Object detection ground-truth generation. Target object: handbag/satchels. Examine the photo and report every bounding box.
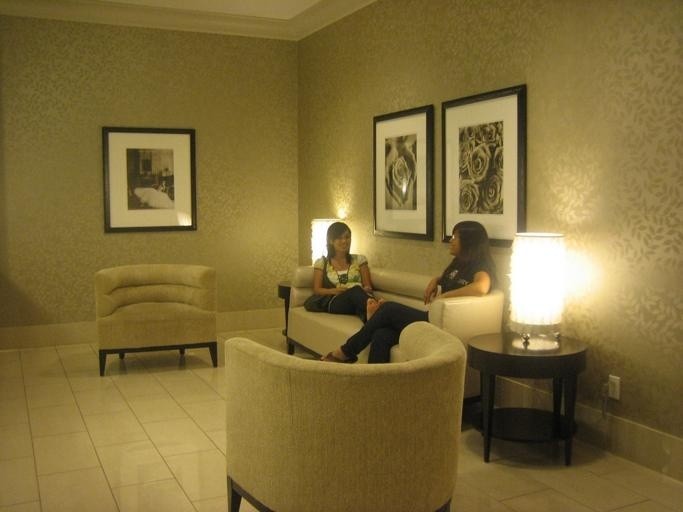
[304,280,336,312]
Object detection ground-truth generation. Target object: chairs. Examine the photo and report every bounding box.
[224,321,466,511]
[93,264,217,377]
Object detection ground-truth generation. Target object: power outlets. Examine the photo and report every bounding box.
[606,375,621,401]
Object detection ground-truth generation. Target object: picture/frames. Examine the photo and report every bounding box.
[372,83,526,249]
[100,125,198,233]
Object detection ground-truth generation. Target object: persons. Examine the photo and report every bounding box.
[318,220,499,368]
[312,221,386,326]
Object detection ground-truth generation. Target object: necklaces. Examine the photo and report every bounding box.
[333,261,349,286]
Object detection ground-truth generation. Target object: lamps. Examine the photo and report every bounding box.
[310,218,339,266]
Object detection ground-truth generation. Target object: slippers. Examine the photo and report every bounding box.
[321,352,357,363]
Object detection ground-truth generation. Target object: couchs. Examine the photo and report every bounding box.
[286,265,505,423]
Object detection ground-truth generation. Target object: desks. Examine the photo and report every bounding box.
[466,333,588,467]
